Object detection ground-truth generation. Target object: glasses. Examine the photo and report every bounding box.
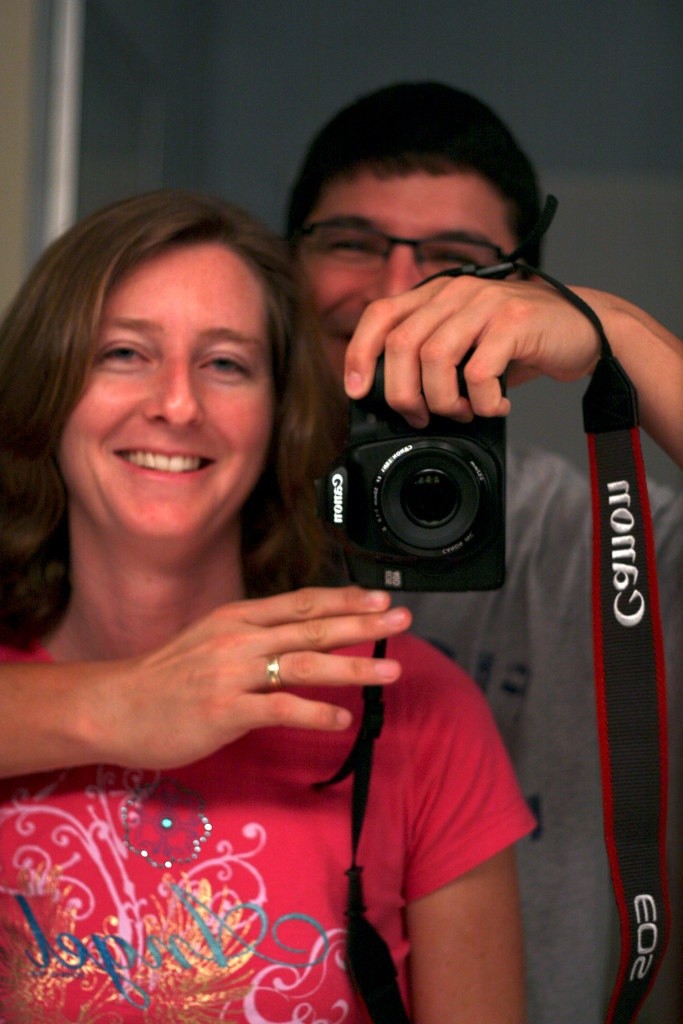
[286,223,515,282]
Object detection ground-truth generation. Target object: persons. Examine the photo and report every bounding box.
[0,80,683,1024]
[0,189,537,1024]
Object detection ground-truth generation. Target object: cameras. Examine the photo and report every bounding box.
[316,354,509,592]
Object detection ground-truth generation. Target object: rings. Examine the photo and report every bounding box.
[262,649,283,695]
